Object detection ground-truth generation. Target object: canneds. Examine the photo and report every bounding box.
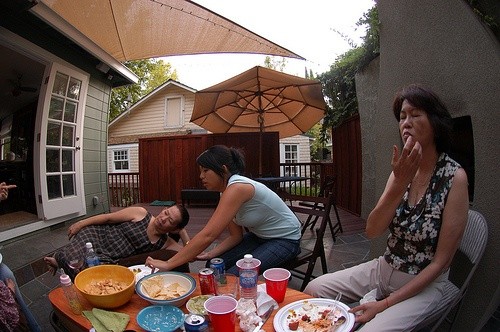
[199,268,217,296]
[210,258,227,287]
[184,314,208,332]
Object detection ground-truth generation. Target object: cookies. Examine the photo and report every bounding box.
[84,279,123,294]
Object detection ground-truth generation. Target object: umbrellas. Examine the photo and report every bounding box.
[191,65,326,178]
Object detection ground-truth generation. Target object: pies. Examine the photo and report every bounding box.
[141,275,188,300]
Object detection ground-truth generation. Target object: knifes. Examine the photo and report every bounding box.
[244,304,274,332]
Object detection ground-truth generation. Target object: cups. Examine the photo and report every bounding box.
[235,258,261,298]
[204,295,238,332]
[263,268,292,303]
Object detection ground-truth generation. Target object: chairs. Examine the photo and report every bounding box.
[301,171,342,234]
[427,210,488,332]
[277,188,334,292]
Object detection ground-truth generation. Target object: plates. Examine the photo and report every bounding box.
[127,263,160,286]
[185,295,215,315]
[272,297,355,332]
[136,305,185,332]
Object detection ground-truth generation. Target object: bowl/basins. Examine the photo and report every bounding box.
[74,265,136,309]
[135,271,197,307]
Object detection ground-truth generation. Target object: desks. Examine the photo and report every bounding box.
[255,176,307,185]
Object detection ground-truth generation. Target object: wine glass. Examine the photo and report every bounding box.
[62,241,84,274]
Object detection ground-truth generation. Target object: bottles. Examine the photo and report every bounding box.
[58,268,82,315]
[238,254,258,303]
[84,242,100,268]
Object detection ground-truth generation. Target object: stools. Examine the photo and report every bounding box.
[117,246,190,272]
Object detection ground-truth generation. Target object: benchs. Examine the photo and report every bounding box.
[179,188,222,207]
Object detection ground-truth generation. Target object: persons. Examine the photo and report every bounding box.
[303,85,470,332]
[0,182,42,332]
[145,145,301,277]
[44,203,190,283]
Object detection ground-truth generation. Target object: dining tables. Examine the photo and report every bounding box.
[48,272,314,332]
